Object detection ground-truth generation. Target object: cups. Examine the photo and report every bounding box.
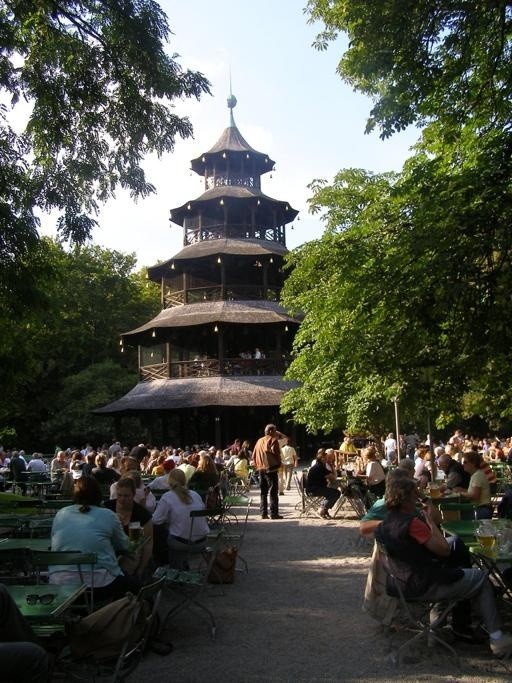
[476,530,495,550]
[128,521,141,543]
[430,485,440,498]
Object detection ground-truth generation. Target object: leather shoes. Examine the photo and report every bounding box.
[271,515,284,520]
[262,514,270,519]
[487,633,512,655]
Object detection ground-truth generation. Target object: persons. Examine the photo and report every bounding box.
[250,424,285,520]
[152,456,166,476]
[52,441,96,490]
[104,479,155,590]
[152,468,211,571]
[207,446,217,462]
[183,446,193,457]
[110,458,157,511]
[1,447,50,498]
[120,443,149,479]
[228,438,241,456]
[392,460,428,502]
[144,449,160,475]
[187,455,220,504]
[454,454,494,520]
[222,448,233,461]
[359,468,477,642]
[144,459,175,491]
[375,478,512,659]
[279,438,297,495]
[225,450,249,493]
[160,443,187,466]
[339,428,512,462]
[49,475,135,604]
[190,445,219,487]
[304,452,342,519]
[325,447,338,477]
[353,444,385,498]
[213,448,225,464]
[237,440,250,465]
[435,454,472,491]
[97,438,129,458]
[92,453,120,499]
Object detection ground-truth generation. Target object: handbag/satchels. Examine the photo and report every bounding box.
[201,532,239,585]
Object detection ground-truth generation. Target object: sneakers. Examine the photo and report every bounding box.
[279,492,285,496]
[320,511,330,518]
[286,485,291,490]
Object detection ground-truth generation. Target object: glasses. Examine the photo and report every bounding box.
[23,592,60,607]
[460,459,473,465]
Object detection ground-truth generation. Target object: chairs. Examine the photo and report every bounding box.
[148,551,216,644]
[172,508,226,596]
[77,572,172,683]
[438,501,476,544]
[231,468,253,496]
[207,499,253,574]
[373,531,465,665]
[290,467,332,519]
